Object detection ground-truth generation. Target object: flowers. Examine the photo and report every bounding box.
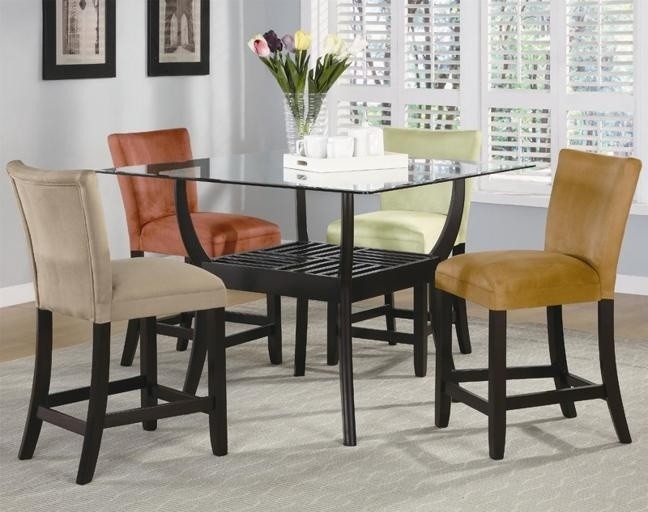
[247,29,368,134]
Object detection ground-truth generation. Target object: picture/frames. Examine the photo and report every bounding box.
[42,0,117,80]
[147,0,211,77]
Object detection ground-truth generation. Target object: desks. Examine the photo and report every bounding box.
[94,153,539,449]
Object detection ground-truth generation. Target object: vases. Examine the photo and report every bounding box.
[283,92,328,152]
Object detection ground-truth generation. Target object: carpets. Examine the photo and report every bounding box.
[0,297,648,512]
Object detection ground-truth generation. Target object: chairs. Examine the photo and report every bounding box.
[433,148,641,462]
[107,127,283,367]
[8,160,228,486]
[325,126,483,376]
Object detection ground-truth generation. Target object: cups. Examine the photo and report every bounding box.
[296,124,384,160]
